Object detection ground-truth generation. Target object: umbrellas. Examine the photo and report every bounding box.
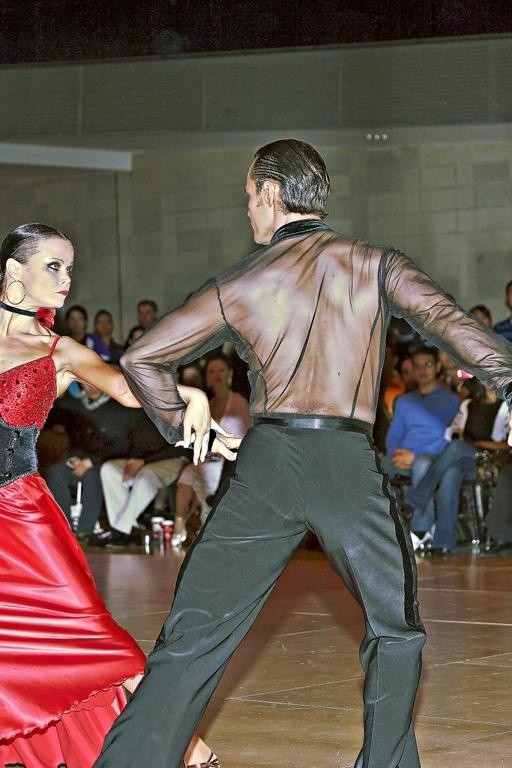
[87,528,189,547]
[398,503,512,558]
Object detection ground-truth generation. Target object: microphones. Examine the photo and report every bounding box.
[385,473,492,556]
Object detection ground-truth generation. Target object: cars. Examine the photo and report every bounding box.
[150,515,174,543]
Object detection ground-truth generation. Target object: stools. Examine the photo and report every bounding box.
[253,417,370,434]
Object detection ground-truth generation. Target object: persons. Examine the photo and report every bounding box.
[97,138,511,768]
[1,222,230,768]
[372,281,510,554]
[37,300,252,546]
